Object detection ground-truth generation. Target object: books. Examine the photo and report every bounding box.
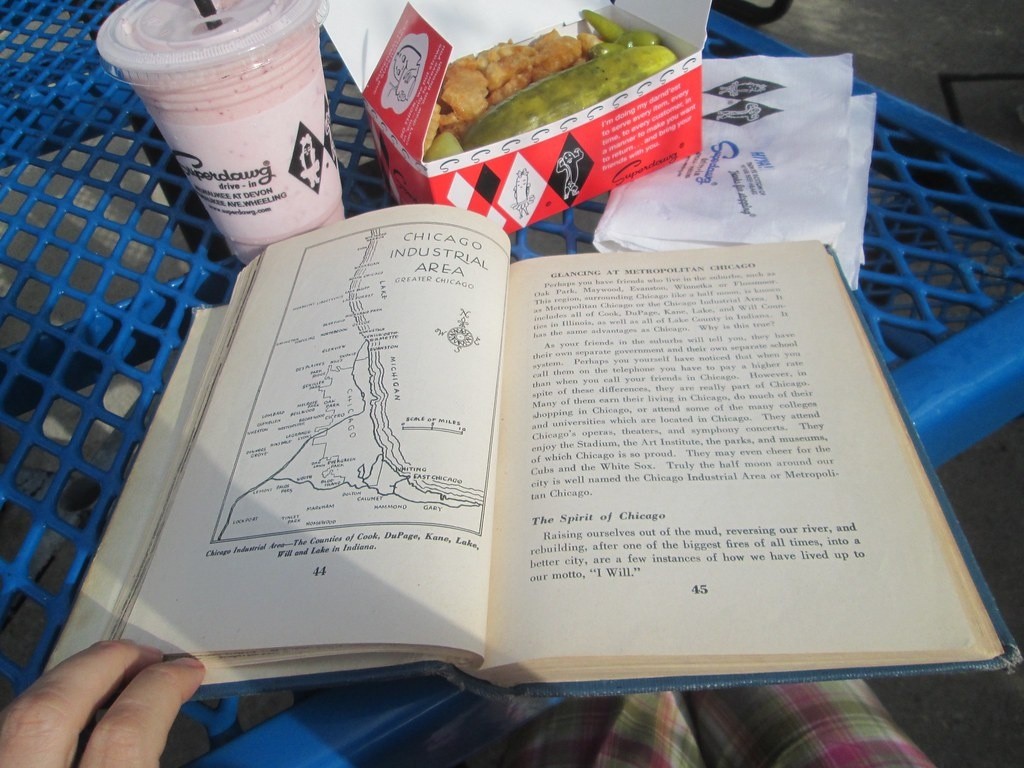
[39,202,1024,704]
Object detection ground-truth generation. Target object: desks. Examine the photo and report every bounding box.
[0,0,1024,768]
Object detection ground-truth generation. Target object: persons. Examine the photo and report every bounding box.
[0,634,941,768]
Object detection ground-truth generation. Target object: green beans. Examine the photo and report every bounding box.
[581,9,660,61]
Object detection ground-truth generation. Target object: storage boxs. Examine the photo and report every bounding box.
[322,0,713,235]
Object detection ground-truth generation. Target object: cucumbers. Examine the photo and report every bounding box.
[458,43,682,153]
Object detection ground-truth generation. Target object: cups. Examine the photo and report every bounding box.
[95,0,346,266]
